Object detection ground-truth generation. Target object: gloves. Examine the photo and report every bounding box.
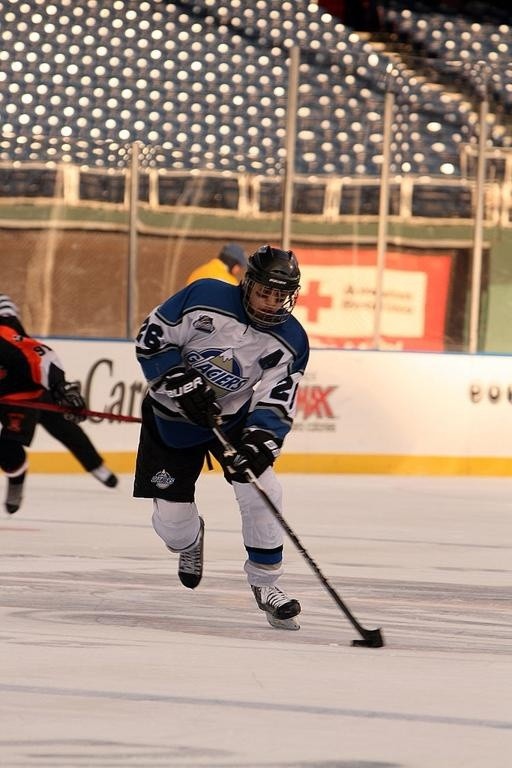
[163,369,227,431]
[221,431,280,484]
[59,383,89,423]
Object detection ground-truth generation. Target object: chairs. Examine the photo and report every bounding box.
[0,1,512,223]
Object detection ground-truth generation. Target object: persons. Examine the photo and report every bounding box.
[132,243,309,619]
[1,292,118,512]
[187,243,248,288]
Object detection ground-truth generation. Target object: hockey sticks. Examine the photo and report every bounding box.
[213,428,390,647]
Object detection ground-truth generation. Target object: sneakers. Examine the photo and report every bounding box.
[90,462,119,489]
[177,517,205,589]
[4,474,26,514]
[251,583,302,619]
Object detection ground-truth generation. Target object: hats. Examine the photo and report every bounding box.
[220,241,247,268]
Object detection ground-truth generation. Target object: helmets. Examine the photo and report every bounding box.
[242,244,302,329]
